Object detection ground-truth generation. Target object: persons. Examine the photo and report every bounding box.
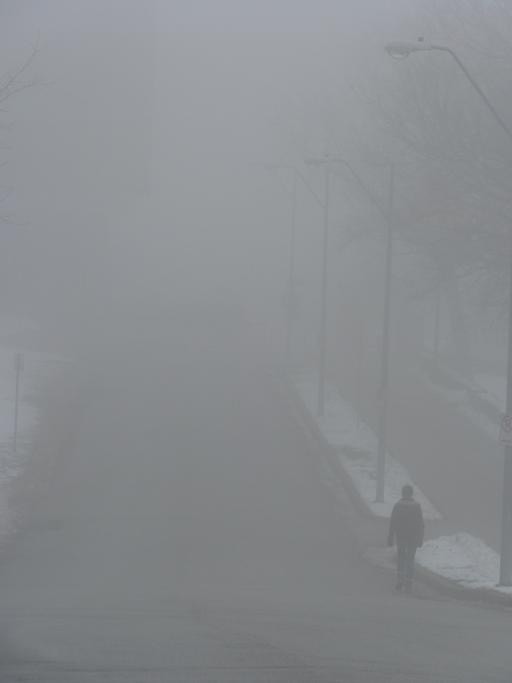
[387,486,425,588]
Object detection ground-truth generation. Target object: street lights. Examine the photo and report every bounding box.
[303,153,396,506]
